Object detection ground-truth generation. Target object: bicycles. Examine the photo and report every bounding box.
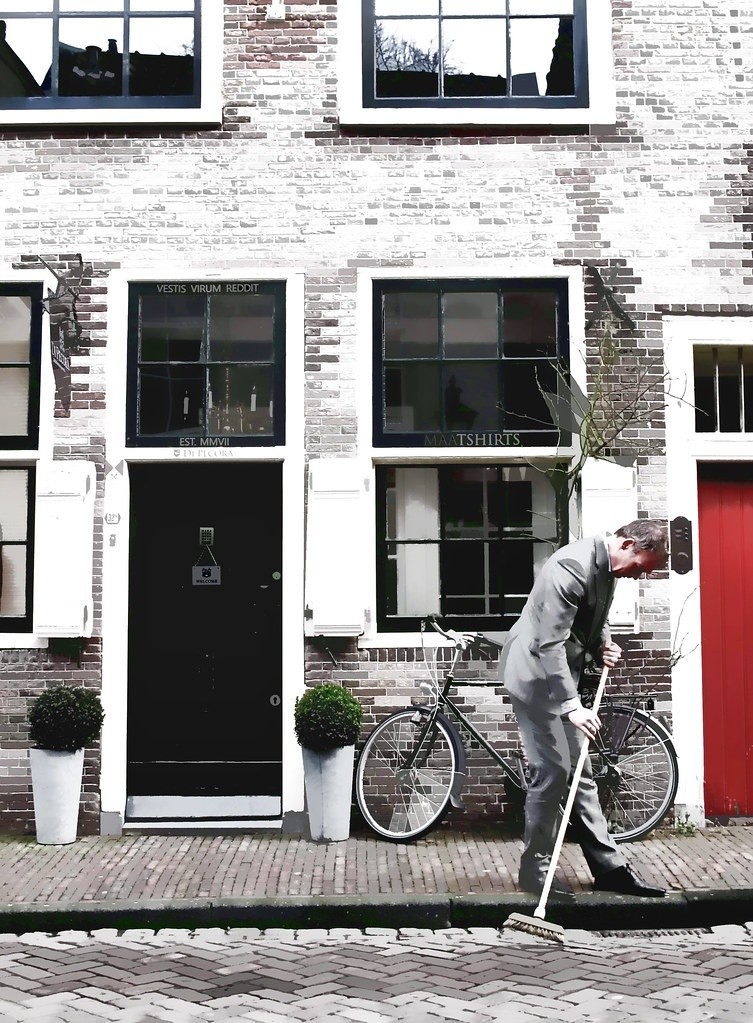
[354,612,681,844]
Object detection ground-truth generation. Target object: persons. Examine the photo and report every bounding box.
[494,517,669,903]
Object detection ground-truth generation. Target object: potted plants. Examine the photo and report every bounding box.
[293,682,364,842]
[26,684,107,844]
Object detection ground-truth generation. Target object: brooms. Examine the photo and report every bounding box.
[501,664,614,947]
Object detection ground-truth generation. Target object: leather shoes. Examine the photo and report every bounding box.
[516,879,578,902]
[593,863,668,897]
